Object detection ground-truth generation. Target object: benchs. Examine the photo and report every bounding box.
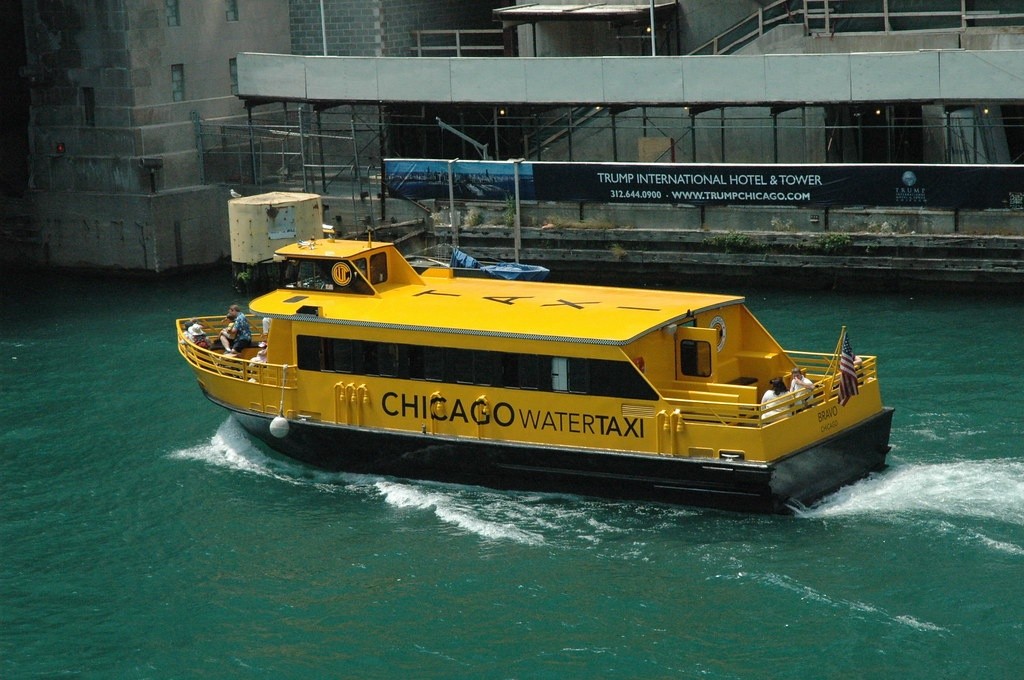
[208,340,267,351]
[724,377,759,386]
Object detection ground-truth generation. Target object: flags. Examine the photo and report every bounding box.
[838,330,859,407]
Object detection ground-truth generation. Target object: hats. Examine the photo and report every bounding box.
[188,323,204,336]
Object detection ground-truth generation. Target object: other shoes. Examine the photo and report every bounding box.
[223,350,238,355]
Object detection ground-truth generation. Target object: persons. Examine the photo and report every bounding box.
[185,318,211,360]
[853,355,863,385]
[239,317,272,382]
[761,378,795,428]
[218,305,252,367]
[217,314,237,341]
[789,368,815,416]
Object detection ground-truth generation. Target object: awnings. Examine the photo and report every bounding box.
[495,3,677,22]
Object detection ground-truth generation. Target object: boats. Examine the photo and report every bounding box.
[174,235,897,515]
[404,241,551,276]
